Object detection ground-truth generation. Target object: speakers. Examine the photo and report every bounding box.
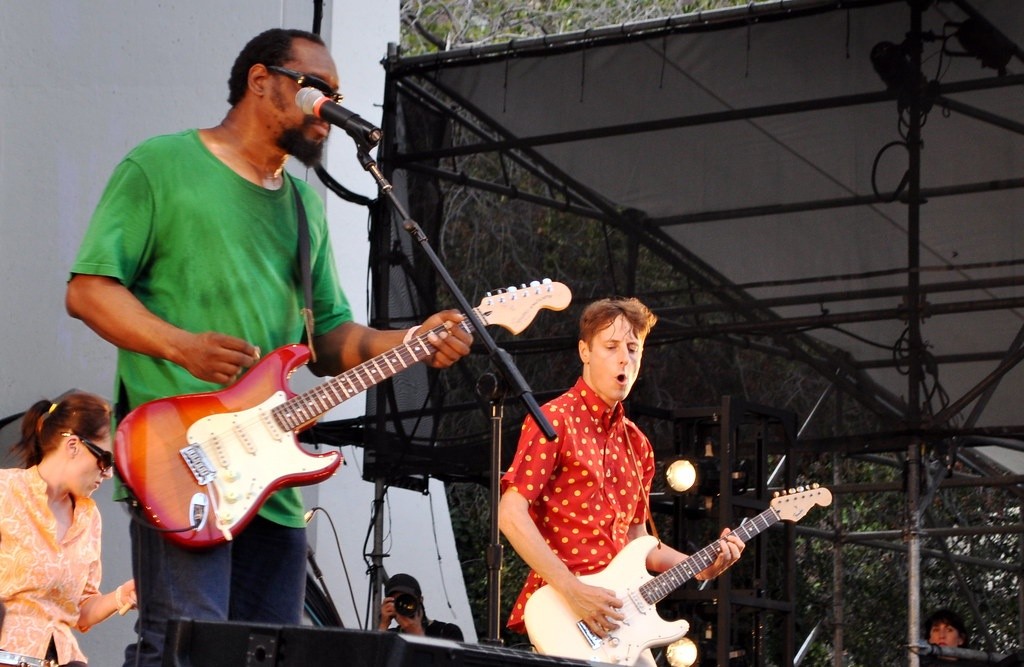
[161,617,626,667]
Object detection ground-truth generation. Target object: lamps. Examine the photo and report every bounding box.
[662,457,718,500]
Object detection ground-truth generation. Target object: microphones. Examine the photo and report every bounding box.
[296,87,381,145]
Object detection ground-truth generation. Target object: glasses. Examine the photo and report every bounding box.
[267,66,343,102]
[60,432,115,472]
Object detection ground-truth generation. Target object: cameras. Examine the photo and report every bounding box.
[393,592,418,617]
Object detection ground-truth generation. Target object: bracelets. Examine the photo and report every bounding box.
[116,585,129,613]
[402,324,421,344]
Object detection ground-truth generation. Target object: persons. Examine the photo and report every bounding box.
[377,573,464,642]
[0,388,112,667]
[63,28,474,667]
[497,295,746,667]
[919,610,969,667]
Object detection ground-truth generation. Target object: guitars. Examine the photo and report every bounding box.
[522,482,833,667]
[112,276,573,550]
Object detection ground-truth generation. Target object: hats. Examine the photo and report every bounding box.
[384,573,421,599]
